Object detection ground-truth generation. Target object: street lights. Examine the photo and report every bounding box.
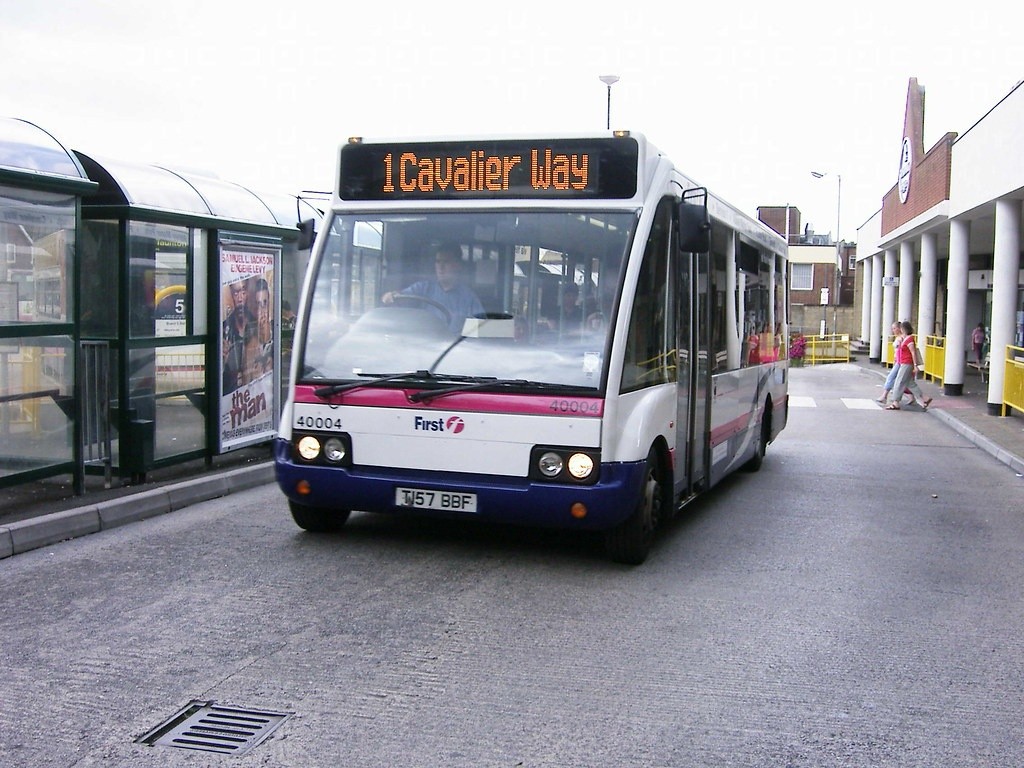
[600,73,620,129]
[811,168,843,359]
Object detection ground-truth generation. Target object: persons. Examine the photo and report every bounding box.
[223,275,275,395]
[383,241,488,339]
[282,300,297,392]
[514,315,528,343]
[745,322,783,367]
[972,323,985,369]
[547,282,605,341]
[876,321,932,410]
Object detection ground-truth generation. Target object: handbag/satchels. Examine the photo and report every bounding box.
[915,348,924,365]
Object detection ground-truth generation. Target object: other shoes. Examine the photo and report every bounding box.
[876,397,887,404]
[906,398,916,405]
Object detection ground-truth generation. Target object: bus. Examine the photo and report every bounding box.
[30,219,249,402]
[275,129,792,565]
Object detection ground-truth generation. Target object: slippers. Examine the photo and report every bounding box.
[922,398,933,409]
[886,404,901,410]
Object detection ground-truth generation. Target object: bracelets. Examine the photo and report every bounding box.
[914,364,917,365]
